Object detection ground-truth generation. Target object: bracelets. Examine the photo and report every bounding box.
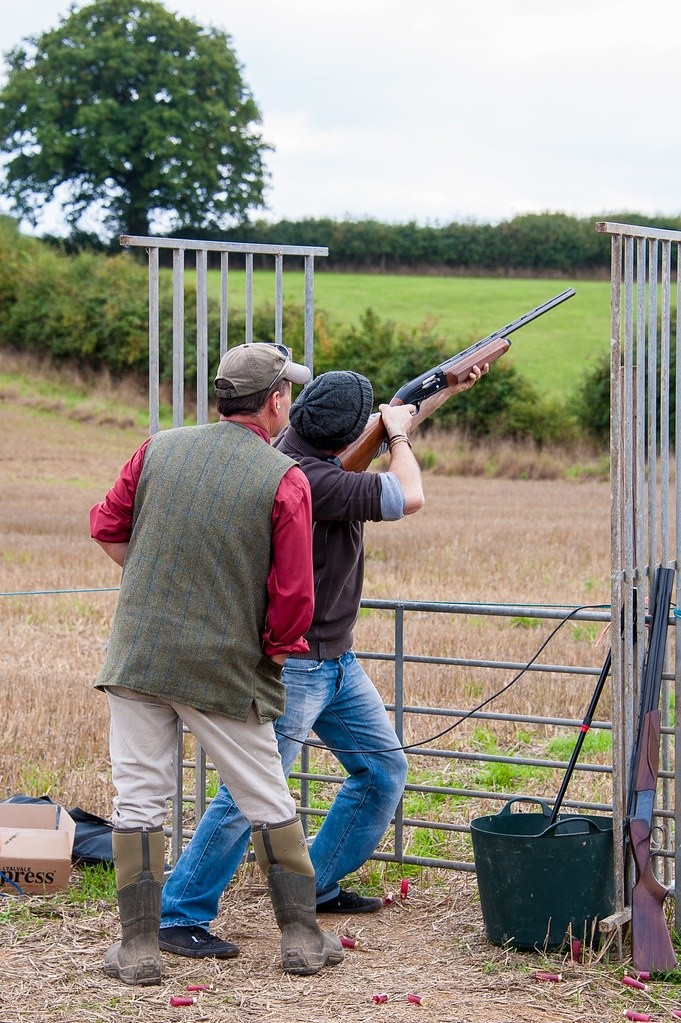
[388,435,413,455]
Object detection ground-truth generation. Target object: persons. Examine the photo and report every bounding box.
[89,341,345,987]
[147,361,491,961]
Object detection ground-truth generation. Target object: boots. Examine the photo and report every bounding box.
[250,815,343,975]
[102,825,165,985]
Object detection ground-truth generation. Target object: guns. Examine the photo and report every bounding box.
[620,567,679,970]
[336,290,575,472]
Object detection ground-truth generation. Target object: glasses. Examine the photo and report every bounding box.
[266,343,290,388]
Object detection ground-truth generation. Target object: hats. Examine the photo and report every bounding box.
[213,342,310,398]
[288,371,372,450]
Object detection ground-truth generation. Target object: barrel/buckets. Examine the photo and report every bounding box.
[470,797,628,954]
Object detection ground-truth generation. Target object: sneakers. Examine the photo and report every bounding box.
[315,890,382,914]
[157,925,240,957]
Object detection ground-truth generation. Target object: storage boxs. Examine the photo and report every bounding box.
[0,803,77,895]
